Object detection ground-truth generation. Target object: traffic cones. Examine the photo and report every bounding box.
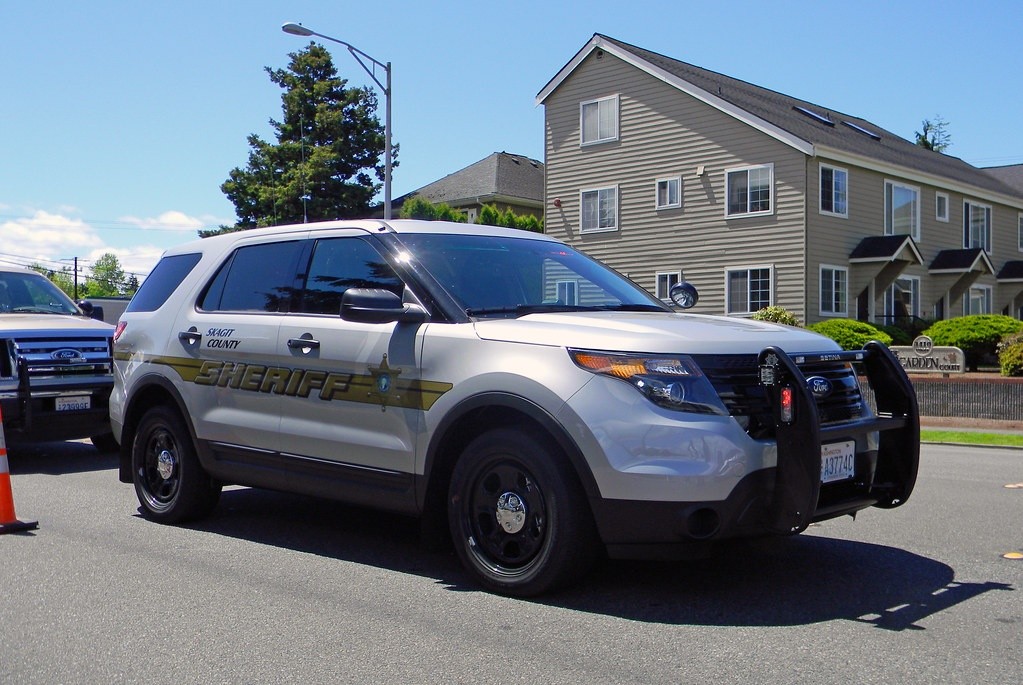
[0,409,40,534]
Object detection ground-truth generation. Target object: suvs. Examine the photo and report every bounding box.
[0,264,121,454]
[108,218,921,600]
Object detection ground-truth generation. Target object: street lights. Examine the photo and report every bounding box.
[282,21,392,220]
